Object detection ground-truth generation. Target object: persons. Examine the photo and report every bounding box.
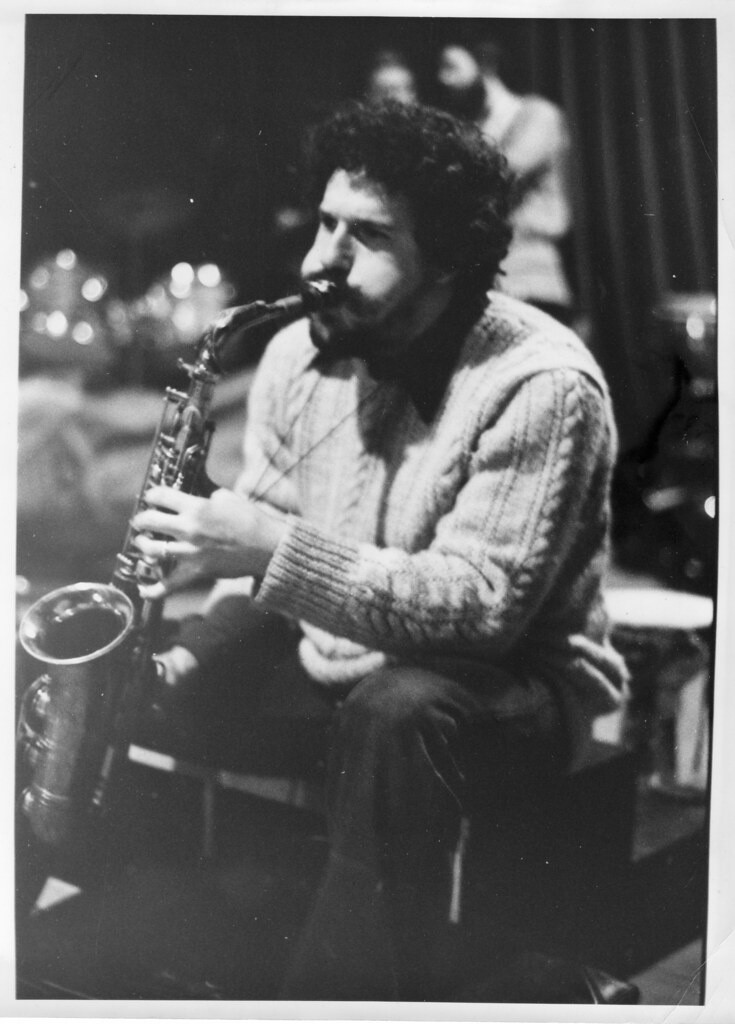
[356,25,572,322]
[130,101,631,1001]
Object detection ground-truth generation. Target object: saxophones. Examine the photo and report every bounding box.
[17,273,347,859]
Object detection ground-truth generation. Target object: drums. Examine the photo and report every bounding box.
[603,587,713,784]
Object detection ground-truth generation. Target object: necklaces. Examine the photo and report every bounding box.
[246,360,401,499]
